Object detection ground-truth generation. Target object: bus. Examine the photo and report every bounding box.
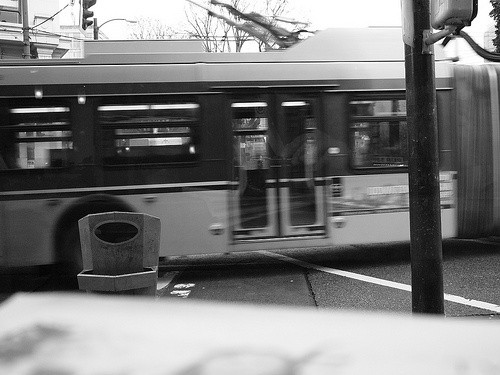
[1,41,500,278]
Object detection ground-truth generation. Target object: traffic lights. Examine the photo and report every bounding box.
[77,0,94,37]
